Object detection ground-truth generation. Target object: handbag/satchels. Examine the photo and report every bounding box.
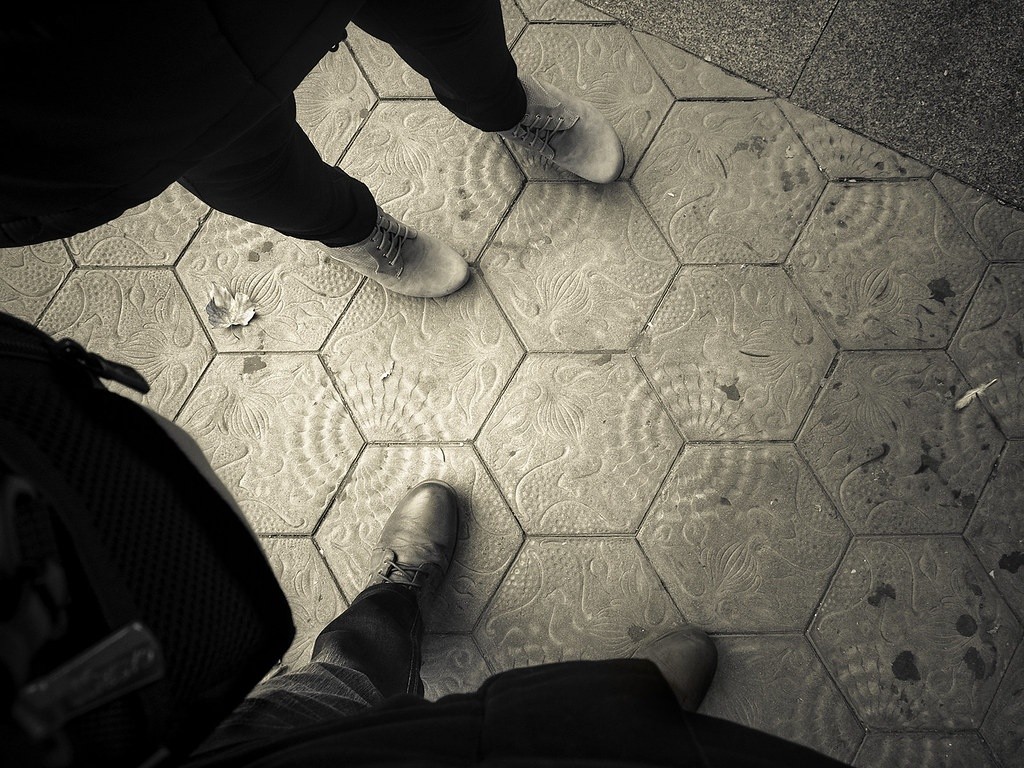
[0,311,295,768]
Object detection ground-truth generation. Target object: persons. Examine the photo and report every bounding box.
[155,479,716,768]
[0,0,625,298]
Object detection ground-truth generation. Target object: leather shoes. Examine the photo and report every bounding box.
[365,479,460,618]
[631,627,718,714]
[309,205,470,298]
[496,70,625,185]
